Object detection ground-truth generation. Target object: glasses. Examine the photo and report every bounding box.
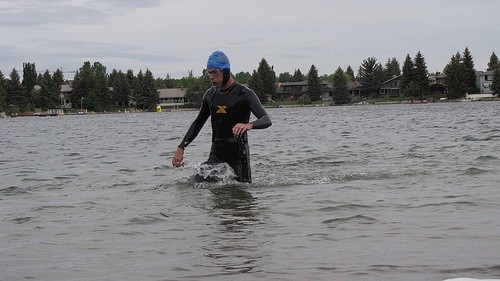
[206,70,221,76]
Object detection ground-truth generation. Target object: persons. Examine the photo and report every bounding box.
[172,50,272,183]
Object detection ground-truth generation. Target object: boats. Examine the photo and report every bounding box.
[8,108,86,118]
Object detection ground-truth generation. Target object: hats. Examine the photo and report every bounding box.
[206,49,230,69]
